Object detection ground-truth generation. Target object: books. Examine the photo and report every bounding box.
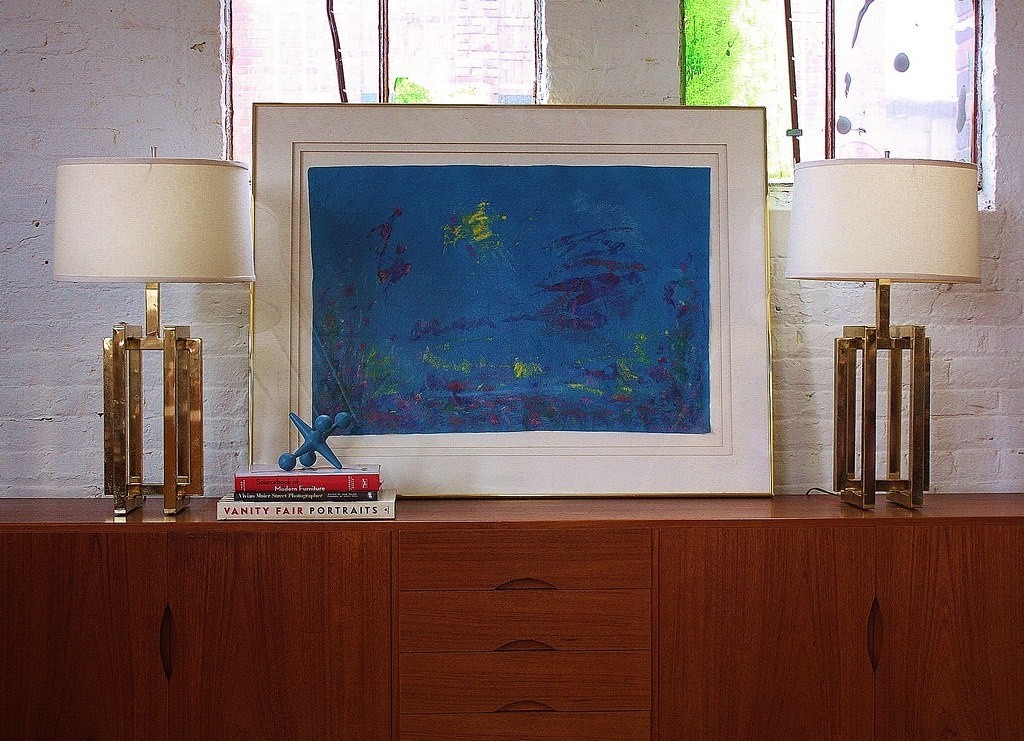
[217,465,397,520]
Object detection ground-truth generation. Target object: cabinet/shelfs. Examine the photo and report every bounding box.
[0,497,1024,741]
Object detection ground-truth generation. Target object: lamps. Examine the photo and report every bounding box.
[52,147,259,516]
[783,150,984,511]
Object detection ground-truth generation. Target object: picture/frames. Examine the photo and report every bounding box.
[245,98,775,497]
